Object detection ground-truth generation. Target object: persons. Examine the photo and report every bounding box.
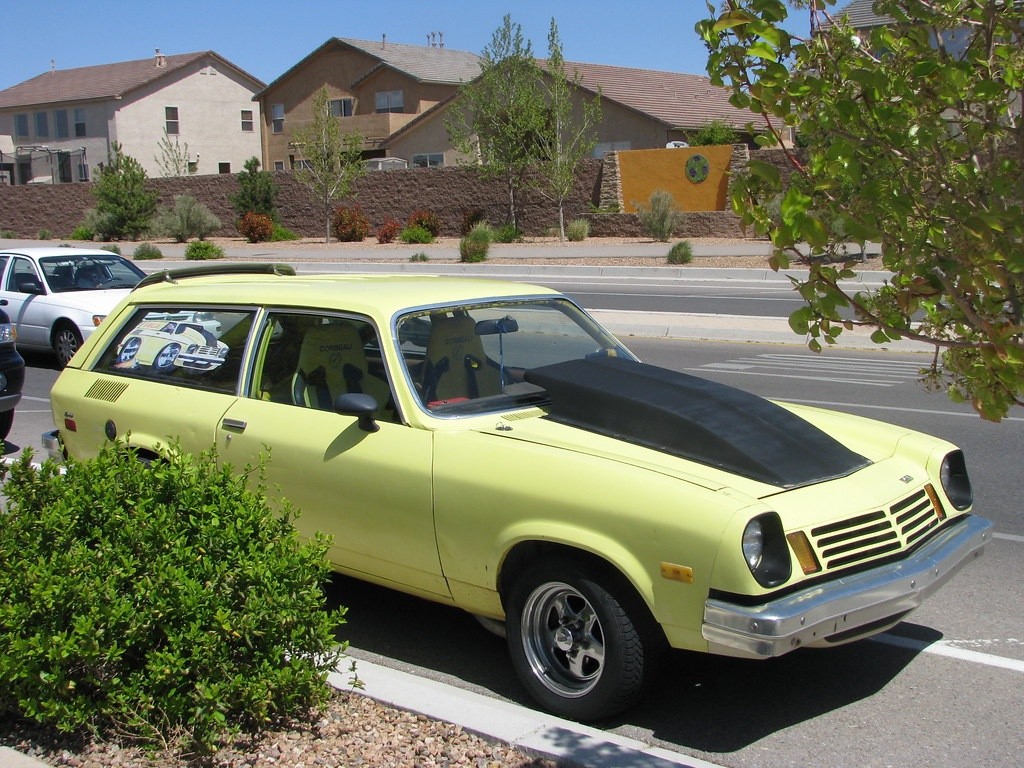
[78,263,105,287]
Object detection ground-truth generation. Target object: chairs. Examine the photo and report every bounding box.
[292,322,395,424]
[421,316,511,407]
[75,265,105,288]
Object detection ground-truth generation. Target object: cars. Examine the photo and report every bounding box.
[0,300,25,445]
[0,246,146,370]
[38,262,995,716]
